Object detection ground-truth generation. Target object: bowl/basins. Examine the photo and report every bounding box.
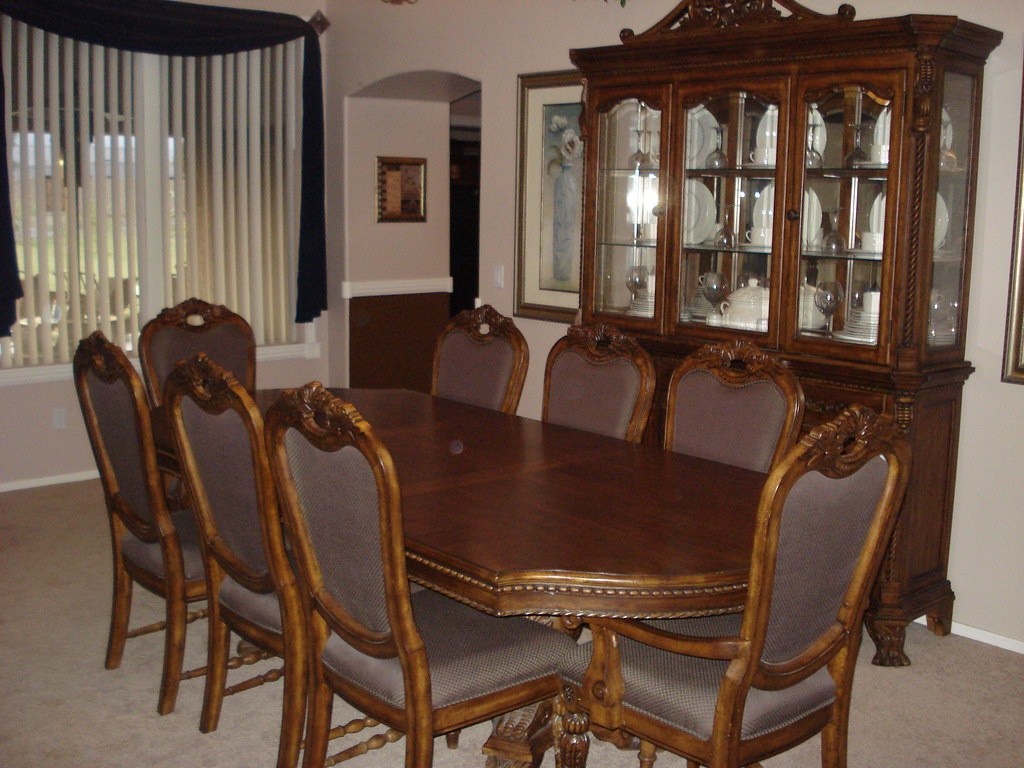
[720,278,770,332]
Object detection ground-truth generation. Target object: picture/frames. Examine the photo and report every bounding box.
[375,156,427,224]
[512,69,584,324]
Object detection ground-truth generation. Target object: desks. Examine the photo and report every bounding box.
[157,388,770,768]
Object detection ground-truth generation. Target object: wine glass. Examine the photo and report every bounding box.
[705,126,728,169]
[702,273,727,313]
[820,207,847,255]
[815,281,844,332]
[938,118,957,172]
[713,203,740,247]
[628,129,649,170]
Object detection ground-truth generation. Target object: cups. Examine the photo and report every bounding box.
[863,292,880,313]
[861,232,883,252]
[869,144,889,163]
[646,275,655,292]
[750,147,776,164]
[746,226,770,246]
[626,266,648,295]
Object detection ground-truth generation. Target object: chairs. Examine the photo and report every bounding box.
[432,304,530,415]
[138,298,257,409]
[164,354,428,768]
[73,331,208,715]
[556,404,912,768]
[263,381,579,768]
[664,336,805,475]
[541,323,656,443]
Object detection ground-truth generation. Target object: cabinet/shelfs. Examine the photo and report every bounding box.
[568,1,1005,669]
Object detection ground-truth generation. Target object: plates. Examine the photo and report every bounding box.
[831,307,879,343]
[873,103,952,150]
[854,161,888,169]
[742,163,774,169]
[752,182,823,245]
[625,290,654,317]
[869,188,949,248]
[685,180,718,245]
[756,106,827,167]
[647,108,721,169]
